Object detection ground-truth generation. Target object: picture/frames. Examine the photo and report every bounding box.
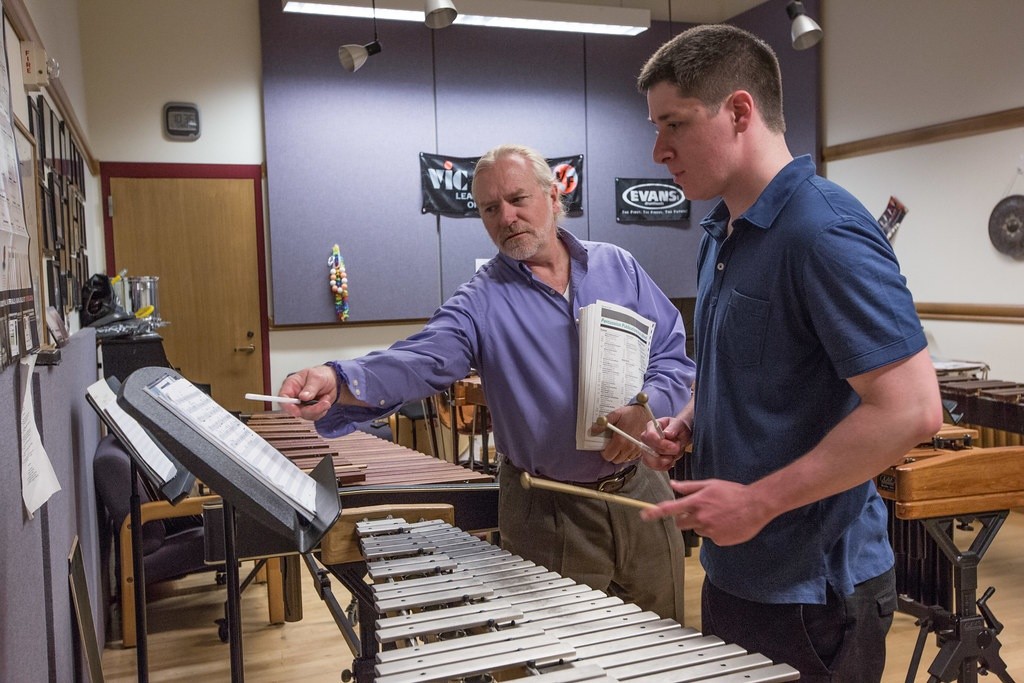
[27,94,88,350]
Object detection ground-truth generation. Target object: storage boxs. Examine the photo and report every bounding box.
[389,412,470,462]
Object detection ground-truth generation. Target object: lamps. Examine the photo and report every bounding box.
[337,0,382,74]
[280,0,652,38]
[783,0,823,51]
[424,0,457,30]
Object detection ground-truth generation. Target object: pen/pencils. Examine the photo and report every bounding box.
[243,393,319,405]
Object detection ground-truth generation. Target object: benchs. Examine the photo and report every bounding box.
[93,433,285,655]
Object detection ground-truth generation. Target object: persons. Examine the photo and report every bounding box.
[280,146,695,627]
[638,26,943,683]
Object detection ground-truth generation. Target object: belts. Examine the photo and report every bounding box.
[506,457,638,494]
